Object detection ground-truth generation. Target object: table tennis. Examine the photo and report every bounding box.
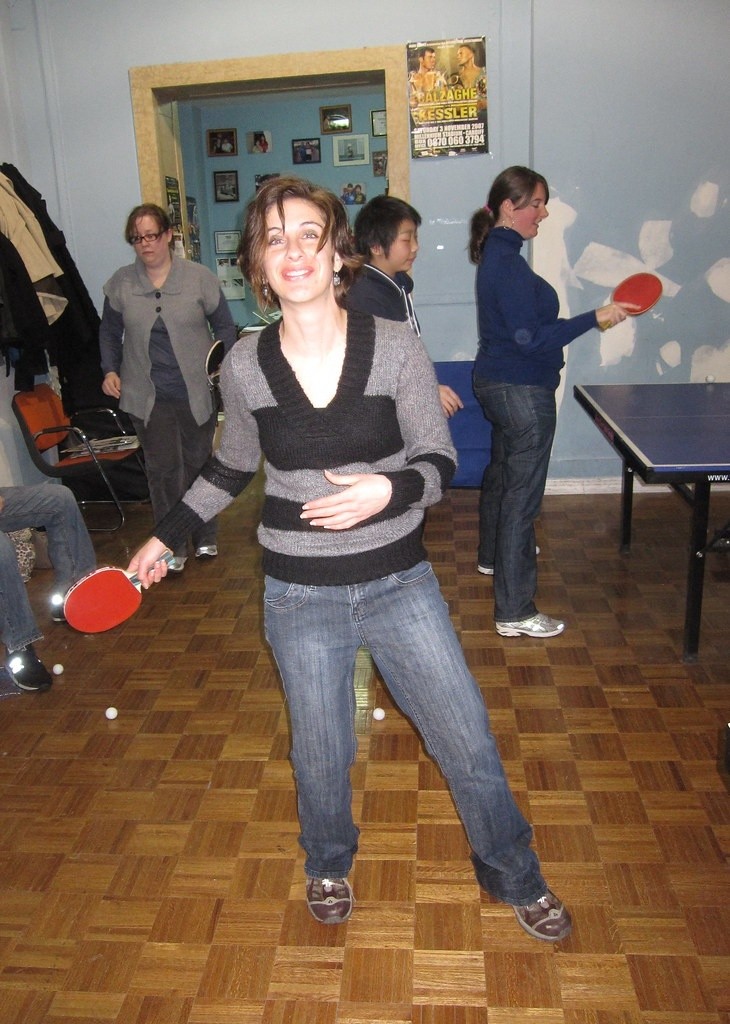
[105,706,118,720]
[373,708,386,721]
[52,664,64,674]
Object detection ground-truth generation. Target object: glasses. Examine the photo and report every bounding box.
[129,229,167,244]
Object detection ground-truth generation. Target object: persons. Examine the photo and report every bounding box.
[0,483,98,685]
[212,137,233,153]
[98,203,237,573]
[299,142,319,162]
[253,134,268,152]
[467,165,642,638]
[338,195,465,421]
[408,44,487,109]
[340,183,366,204]
[128,176,574,941]
[345,144,355,158]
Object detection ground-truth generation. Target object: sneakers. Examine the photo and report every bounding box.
[496,612,567,638]
[194,544,218,558]
[166,553,189,572]
[3,642,53,691]
[478,540,541,575]
[513,888,572,941]
[304,874,353,925]
[49,599,68,622]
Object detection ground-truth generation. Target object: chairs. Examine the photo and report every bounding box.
[11,383,152,536]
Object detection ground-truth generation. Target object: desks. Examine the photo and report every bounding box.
[572,381,730,665]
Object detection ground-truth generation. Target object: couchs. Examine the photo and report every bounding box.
[434,358,493,491]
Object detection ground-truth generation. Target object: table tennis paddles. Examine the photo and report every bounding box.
[599,273,663,332]
[63,551,176,636]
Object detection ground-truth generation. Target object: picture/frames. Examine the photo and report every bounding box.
[212,170,240,203]
[216,257,246,300]
[370,109,387,138]
[331,134,370,167]
[213,230,242,254]
[319,104,352,135]
[291,138,321,164]
[205,127,238,157]
